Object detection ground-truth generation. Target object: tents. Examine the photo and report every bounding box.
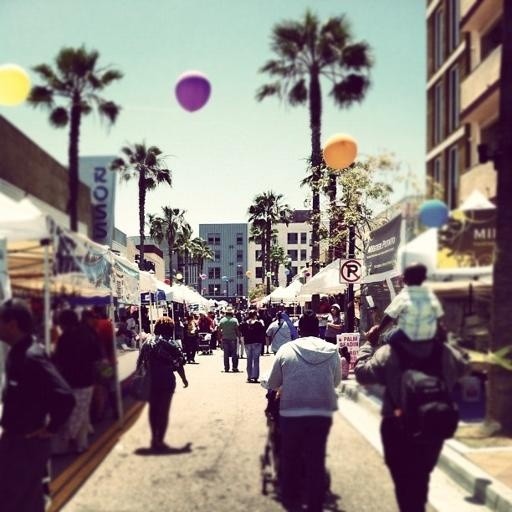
[110,253,233,351]
[253,279,330,317]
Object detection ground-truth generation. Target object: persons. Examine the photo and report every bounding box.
[261,310,342,512]
[218,306,241,373]
[354,327,459,511]
[316,292,349,380]
[1,296,117,512]
[242,310,267,383]
[114,303,300,364]
[136,317,189,451]
[375,263,445,342]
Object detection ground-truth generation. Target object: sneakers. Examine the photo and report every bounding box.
[150,441,169,450]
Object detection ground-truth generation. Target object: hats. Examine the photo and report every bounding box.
[222,307,233,314]
[329,303,341,310]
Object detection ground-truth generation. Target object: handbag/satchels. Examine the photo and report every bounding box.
[129,339,153,401]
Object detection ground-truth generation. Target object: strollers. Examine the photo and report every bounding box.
[257,388,333,502]
[197,333,212,355]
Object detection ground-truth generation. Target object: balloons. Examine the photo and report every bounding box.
[419,199,449,228]
[0,63,31,107]
[174,71,211,112]
[321,135,358,170]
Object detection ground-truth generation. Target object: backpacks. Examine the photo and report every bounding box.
[385,342,461,449]
